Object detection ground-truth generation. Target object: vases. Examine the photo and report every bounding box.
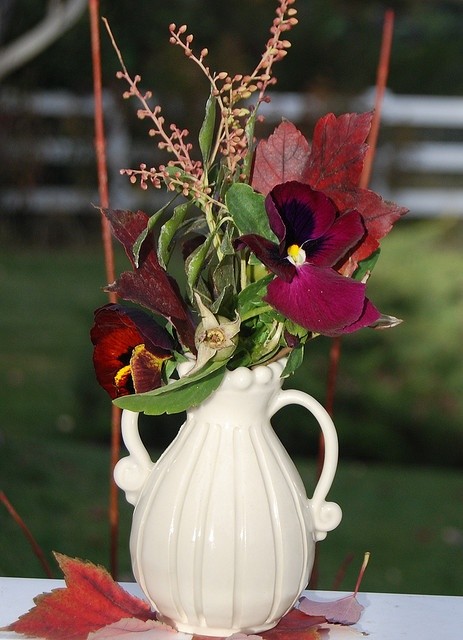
[111,352,343,636]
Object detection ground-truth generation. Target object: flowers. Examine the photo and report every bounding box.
[89,2,407,416]
